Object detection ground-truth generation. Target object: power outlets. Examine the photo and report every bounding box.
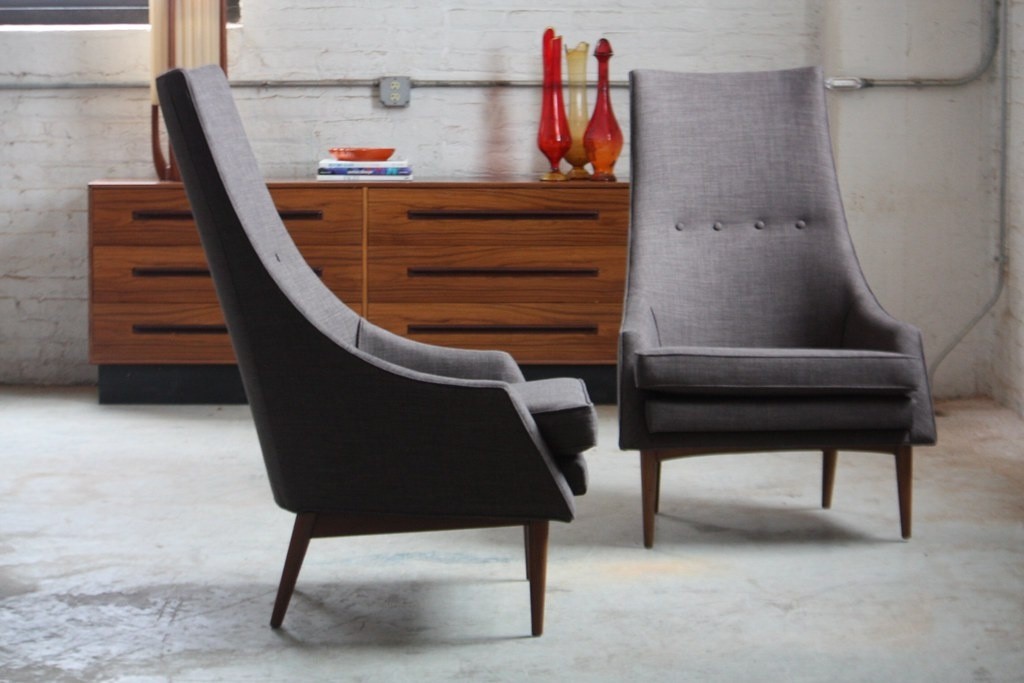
[379,76,411,106]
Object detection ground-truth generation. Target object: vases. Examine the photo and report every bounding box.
[538,28,624,181]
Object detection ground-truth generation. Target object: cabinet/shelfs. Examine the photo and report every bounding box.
[88,181,630,405]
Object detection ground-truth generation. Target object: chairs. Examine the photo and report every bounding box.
[156,64,600,636]
[616,67,938,549]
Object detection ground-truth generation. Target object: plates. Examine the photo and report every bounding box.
[328,147,395,161]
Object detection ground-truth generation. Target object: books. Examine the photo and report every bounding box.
[315,158,414,181]
